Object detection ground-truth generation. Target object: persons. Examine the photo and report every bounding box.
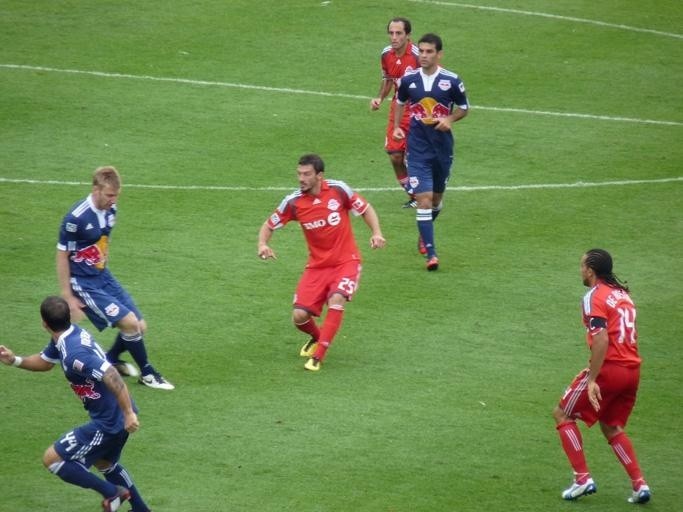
[257,155,387,371]
[368,17,439,210]
[0,297,151,512]
[552,250,650,504]
[54,166,176,390]
[392,32,470,270]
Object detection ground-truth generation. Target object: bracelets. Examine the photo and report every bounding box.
[11,355,23,368]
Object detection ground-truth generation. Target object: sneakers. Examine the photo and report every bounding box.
[403,200,416,208]
[562,478,596,500]
[426,256,439,270]
[139,374,174,390]
[103,485,130,512]
[300,338,319,357]
[304,357,321,370]
[627,484,650,503]
[417,235,428,254]
[115,361,137,376]
